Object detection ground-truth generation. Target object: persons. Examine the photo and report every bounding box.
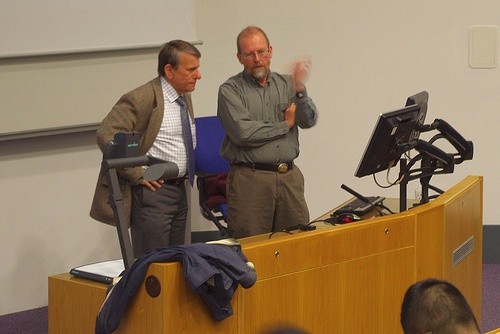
[89,40,202,260]
[217,25,318,238]
[400,278,481,334]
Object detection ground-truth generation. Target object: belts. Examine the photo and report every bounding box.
[235,161,295,173]
[166,179,184,186]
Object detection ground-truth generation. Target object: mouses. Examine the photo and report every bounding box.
[336,213,360,222]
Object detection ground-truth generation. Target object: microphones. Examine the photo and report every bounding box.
[341,184,383,216]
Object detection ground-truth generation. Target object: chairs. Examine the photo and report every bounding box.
[194,116,228,236]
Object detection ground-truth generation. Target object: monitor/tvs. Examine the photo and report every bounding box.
[355,91,430,178]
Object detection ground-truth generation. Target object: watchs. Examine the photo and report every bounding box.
[296,89,306,98]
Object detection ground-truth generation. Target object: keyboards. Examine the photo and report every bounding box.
[330,196,386,216]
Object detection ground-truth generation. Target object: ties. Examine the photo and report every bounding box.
[175,96,195,188]
